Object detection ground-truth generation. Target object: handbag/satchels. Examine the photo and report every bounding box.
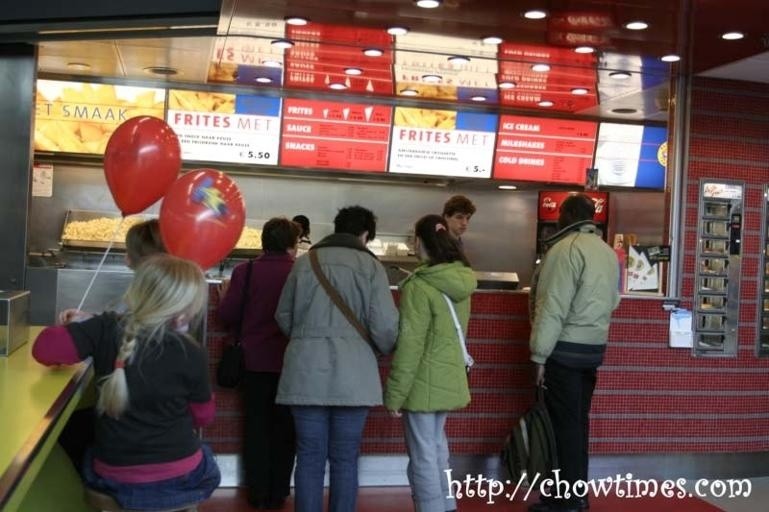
[218,341,243,389]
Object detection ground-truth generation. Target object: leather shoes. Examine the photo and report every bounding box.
[528,492,589,512]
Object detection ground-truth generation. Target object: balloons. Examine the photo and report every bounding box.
[159,167,247,271]
[104,115,181,220]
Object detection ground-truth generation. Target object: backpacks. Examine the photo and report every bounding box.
[501,387,558,490]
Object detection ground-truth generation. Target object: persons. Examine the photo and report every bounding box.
[59,218,188,333]
[32,255,223,512]
[274,204,399,512]
[528,195,621,511]
[211,218,295,512]
[293,215,313,258]
[381,215,478,512]
[442,196,476,266]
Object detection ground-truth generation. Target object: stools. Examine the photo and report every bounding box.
[74,485,211,512]
